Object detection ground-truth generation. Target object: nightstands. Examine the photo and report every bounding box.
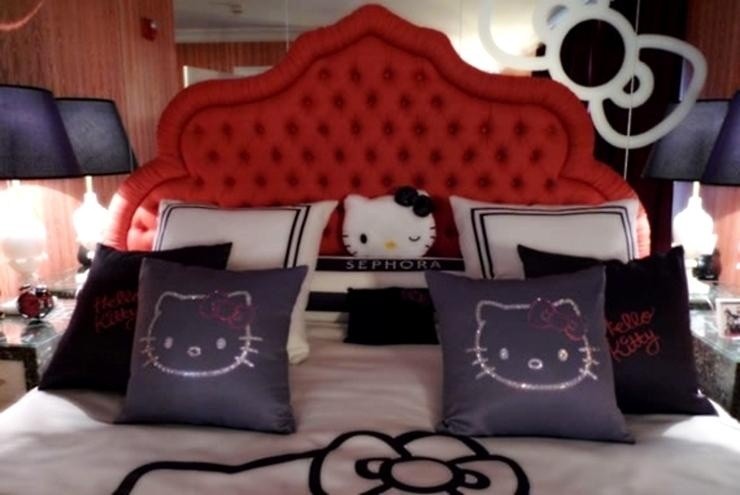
[685,299,740,420]
[0,290,87,391]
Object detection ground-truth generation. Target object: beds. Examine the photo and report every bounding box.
[0,0,740,493]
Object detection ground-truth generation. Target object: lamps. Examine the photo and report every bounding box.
[641,91,739,310]
[1,73,140,324]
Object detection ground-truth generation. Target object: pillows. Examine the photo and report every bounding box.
[152,198,335,364]
[115,258,308,435]
[344,286,439,345]
[515,243,715,415]
[448,197,636,281]
[425,267,636,444]
[38,243,231,395]
